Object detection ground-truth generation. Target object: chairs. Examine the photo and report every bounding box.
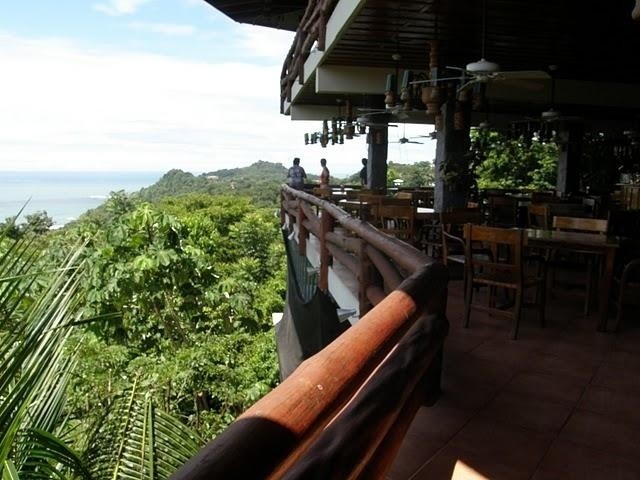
[314,179,440,247]
[414,179,640,343]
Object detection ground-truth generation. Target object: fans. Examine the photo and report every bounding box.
[304,93,398,147]
[355,1,591,148]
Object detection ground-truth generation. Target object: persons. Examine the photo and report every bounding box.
[319,159,329,187]
[287,158,306,188]
[360,158,367,189]
